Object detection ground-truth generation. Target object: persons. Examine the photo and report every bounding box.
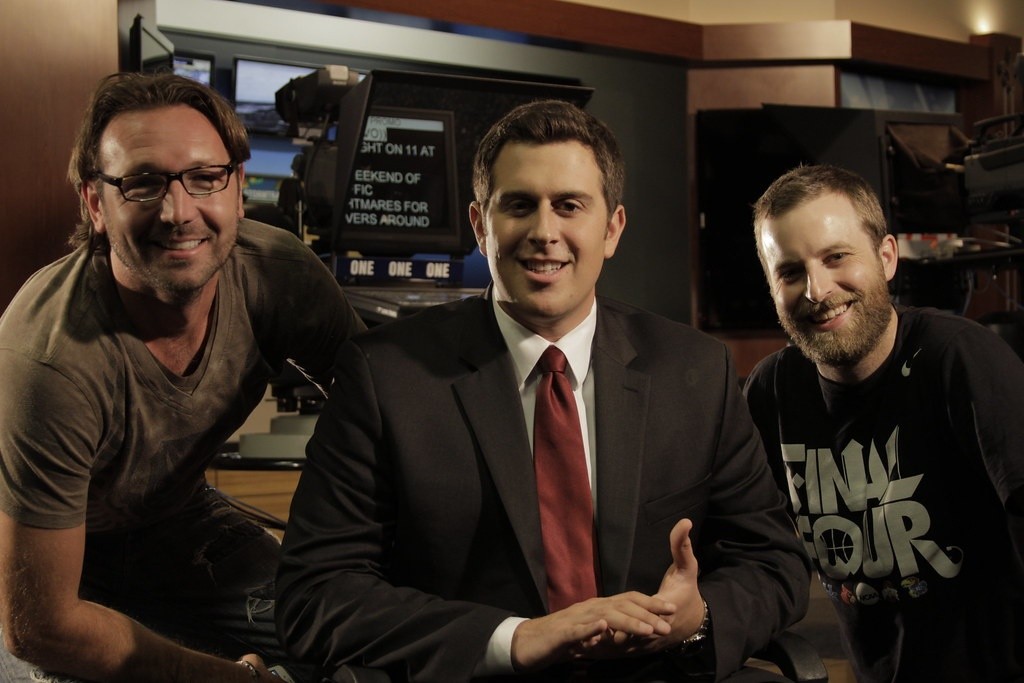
[0,68,374,683]
[741,160,1024,683]
[270,100,812,683]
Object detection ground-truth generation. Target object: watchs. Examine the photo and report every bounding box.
[664,597,712,657]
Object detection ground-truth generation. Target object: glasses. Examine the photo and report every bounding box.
[90,159,238,202]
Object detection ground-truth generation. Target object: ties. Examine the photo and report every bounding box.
[533,344,598,614]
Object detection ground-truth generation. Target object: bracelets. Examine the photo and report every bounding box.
[235,660,263,683]
